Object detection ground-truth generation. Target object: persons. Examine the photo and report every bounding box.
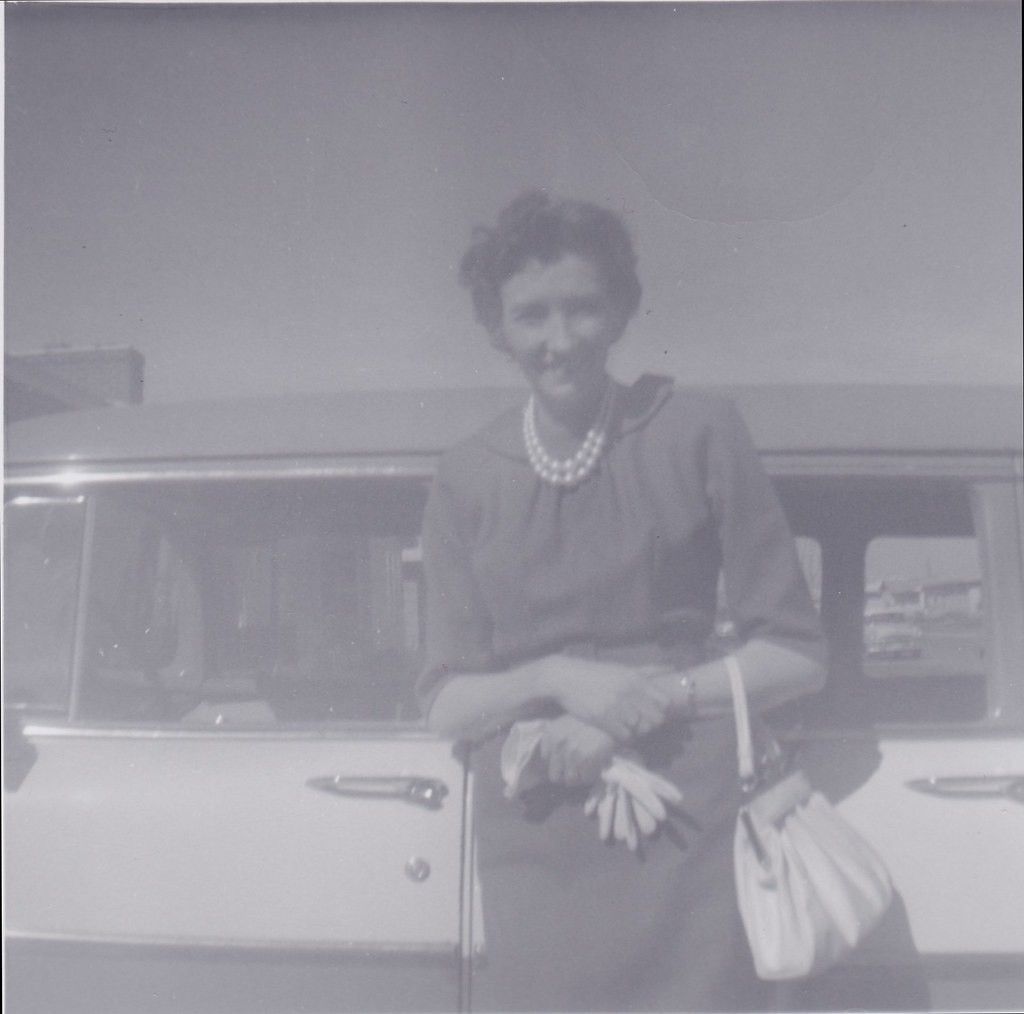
[418,194,826,1014]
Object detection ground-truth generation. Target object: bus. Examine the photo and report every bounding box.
[4,378,1024,969]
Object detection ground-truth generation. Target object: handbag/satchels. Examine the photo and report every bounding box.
[724,656,894,980]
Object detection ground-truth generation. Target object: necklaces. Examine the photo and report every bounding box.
[523,377,613,486]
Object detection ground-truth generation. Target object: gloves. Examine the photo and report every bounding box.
[501,722,683,850]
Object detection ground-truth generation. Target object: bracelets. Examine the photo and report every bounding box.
[677,671,696,721]
